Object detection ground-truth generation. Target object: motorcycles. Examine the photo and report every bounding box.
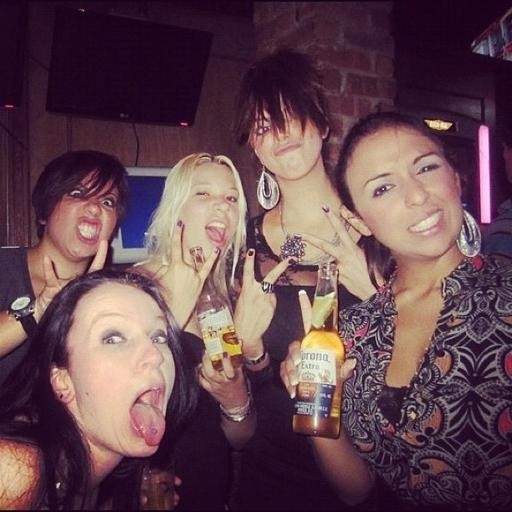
[46,7,214,127]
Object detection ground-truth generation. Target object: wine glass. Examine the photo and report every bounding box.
[261,282,276,294]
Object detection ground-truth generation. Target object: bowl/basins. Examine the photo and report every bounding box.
[277,195,352,267]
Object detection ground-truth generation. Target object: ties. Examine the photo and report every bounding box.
[111,167,172,265]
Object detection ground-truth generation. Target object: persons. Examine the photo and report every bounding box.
[484,120,511,264]
[1,267,187,511]
[133,151,259,510]
[231,51,395,512]
[0,151,133,392]
[279,110,512,512]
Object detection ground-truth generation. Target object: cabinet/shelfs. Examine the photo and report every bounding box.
[189,245,245,373]
[292,259,347,440]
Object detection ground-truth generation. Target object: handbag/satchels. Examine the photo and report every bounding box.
[218,393,255,424]
[246,351,268,366]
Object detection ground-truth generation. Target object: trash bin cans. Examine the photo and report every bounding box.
[7,294,37,338]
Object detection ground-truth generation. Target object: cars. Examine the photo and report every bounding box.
[139,457,176,510]
